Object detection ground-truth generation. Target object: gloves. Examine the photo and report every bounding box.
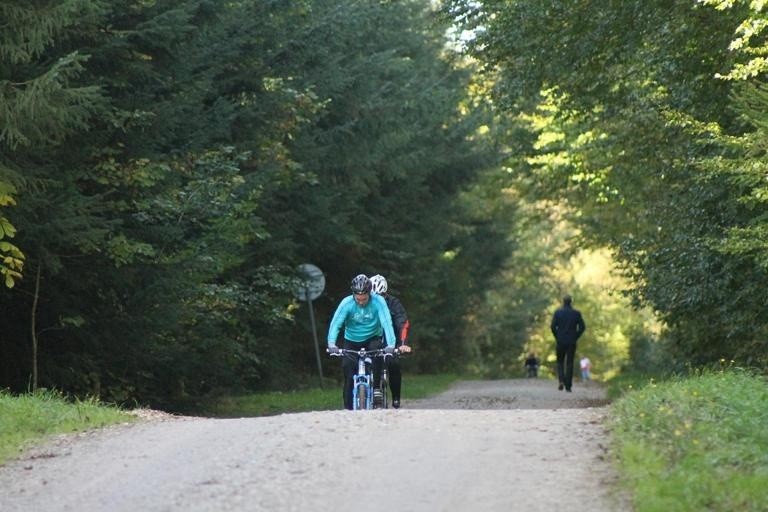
[399,345,411,353]
[384,353,394,367]
[329,347,339,360]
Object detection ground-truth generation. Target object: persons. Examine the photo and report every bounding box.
[551,294,585,392]
[578,354,593,378]
[368,274,410,409]
[526,351,539,378]
[326,272,396,411]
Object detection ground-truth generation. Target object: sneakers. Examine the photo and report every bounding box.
[392,395,400,409]
[558,383,572,392]
[373,389,383,404]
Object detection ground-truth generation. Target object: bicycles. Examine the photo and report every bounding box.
[526,365,538,378]
[325,345,413,410]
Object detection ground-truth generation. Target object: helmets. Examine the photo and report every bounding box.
[351,274,387,294]
